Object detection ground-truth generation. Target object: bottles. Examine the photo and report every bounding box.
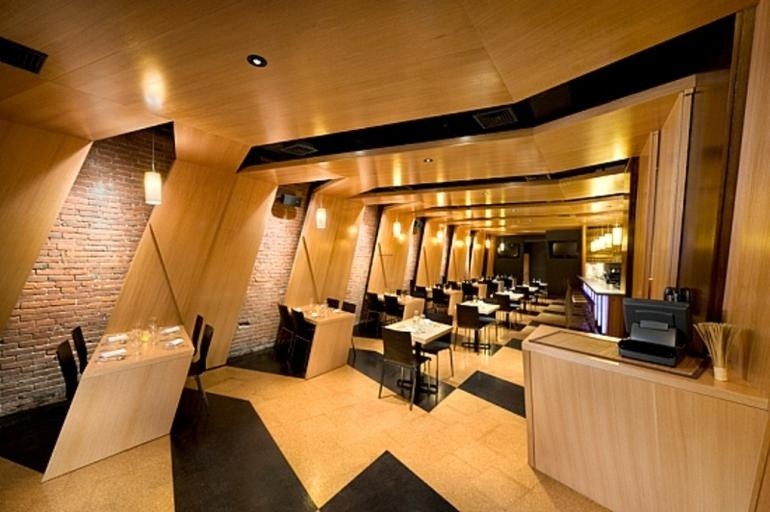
[397,284,408,301]
[472,295,484,310]
[662,285,692,302]
[413,309,426,333]
[100,316,185,362]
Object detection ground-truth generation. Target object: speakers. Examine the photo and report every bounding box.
[415,221,423,228]
[281,194,302,207]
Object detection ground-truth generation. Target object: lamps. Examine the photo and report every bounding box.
[315,194,328,230]
[590,216,624,253]
[143,127,164,205]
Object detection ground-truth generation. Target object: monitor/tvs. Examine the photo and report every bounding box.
[497,242,520,259]
[618,298,694,367]
[547,239,581,259]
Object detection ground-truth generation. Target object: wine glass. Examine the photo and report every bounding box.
[306,297,334,320]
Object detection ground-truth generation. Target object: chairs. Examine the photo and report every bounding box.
[270,272,549,411]
[564,279,595,333]
[40,311,215,485]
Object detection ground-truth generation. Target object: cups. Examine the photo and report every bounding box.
[714,367,728,381]
